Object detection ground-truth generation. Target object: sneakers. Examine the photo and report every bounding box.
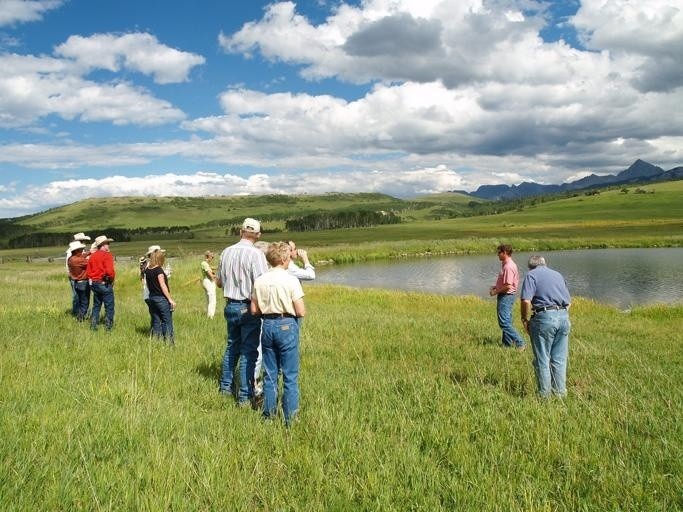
[517,344,527,353]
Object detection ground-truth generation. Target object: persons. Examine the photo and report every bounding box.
[519,255,571,401]
[215,217,269,408]
[64,232,91,285]
[248,241,305,430]
[488,243,527,351]
[138,244,172,335]
[252,318,264,403]
[86,235,115,334]
[282,239,315,285]
[142,249,176,351]
[65,240,93,322]
[198,250,216,319]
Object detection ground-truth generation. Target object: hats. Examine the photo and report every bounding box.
[139,257,146,262]
[65,232,114,254]
[144,245,166,256]
[241,218,261,233]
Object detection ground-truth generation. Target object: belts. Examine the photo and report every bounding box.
[229,299,250,303]
[92,281,109,284]
[76,280,86,283]
[263,314,294,318]
[536,306,566,312]
